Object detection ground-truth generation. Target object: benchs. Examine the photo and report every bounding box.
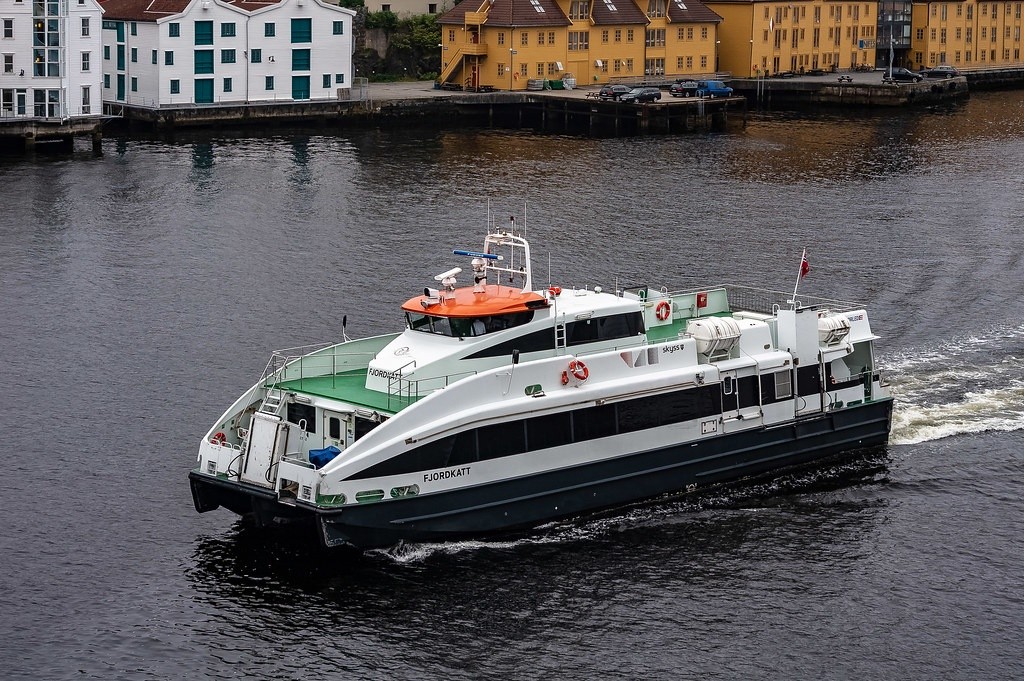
[585,92,600,99]
[881,77,900,84]
[838,75,853,82]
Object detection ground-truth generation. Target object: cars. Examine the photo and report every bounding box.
[917,65,961,79]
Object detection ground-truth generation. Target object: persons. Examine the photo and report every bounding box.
[470,317,486,336]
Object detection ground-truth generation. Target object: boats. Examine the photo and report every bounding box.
[189,194,900,551]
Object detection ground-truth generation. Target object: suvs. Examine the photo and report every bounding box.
[696,81,734,99]
[598,84,634,101]
[619,87,662,104]
[669,80,700,98]
[883,67,924,83]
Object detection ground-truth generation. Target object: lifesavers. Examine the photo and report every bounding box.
[211,433,227,446]
[655,302,670,320]
[570,360,589,379]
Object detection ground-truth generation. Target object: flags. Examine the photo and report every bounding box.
[802,254,809,278]
